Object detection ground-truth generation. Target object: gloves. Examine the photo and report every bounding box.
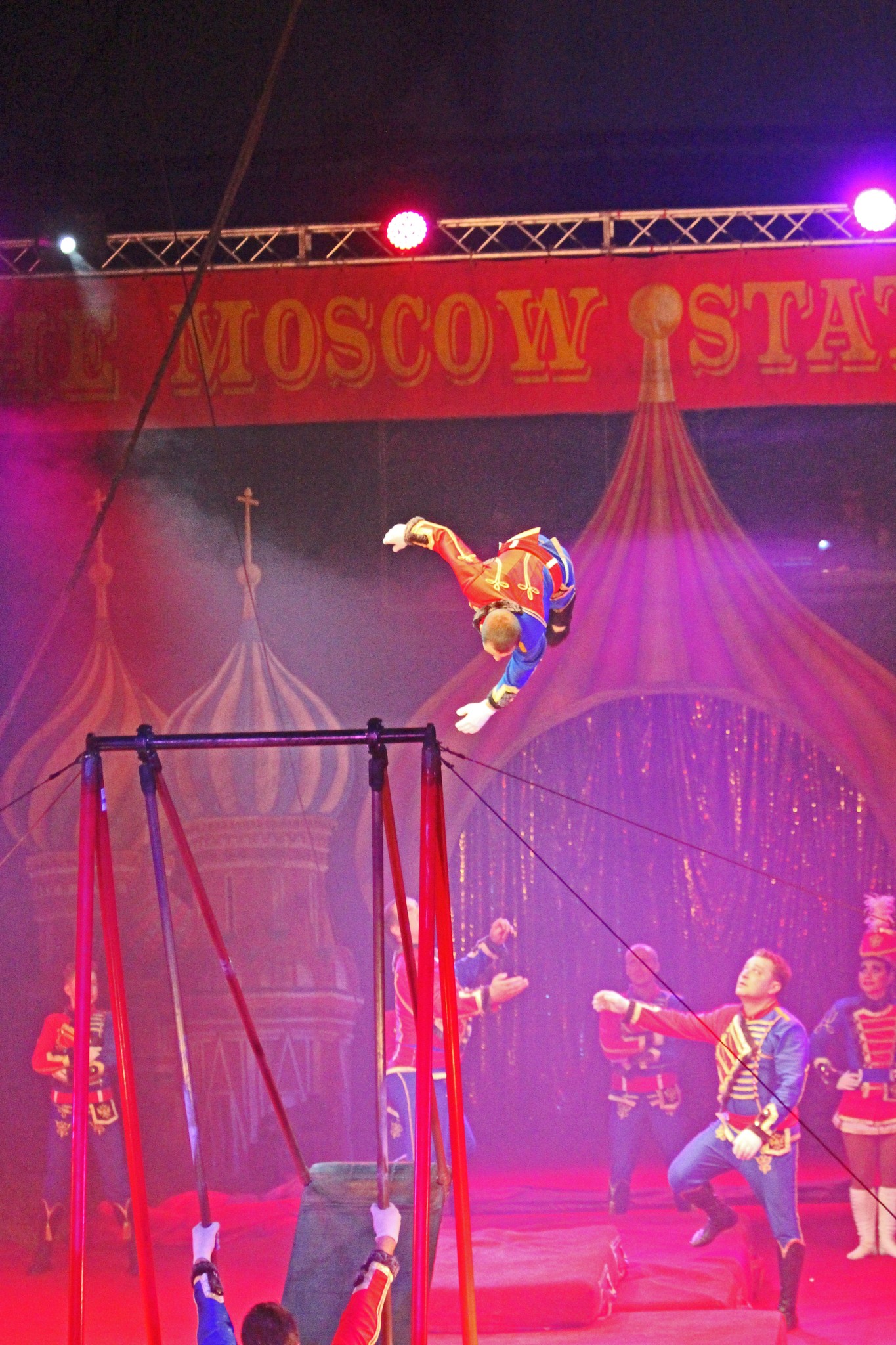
[381,523,407,553]
[191,1220,219,1266]
[731,1132,764,1164]
[834,1071,862,1091]
[454,700,495,736]
[591,989,628,1013]
[370,1202,401,1243]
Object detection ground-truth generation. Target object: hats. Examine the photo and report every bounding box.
[859,893,896,973]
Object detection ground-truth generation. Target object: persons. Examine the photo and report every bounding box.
[27,960,138,1277]
[598,942,697,1216]
[384,516,577,732]
[383,896,529,1164]
[190,1202,402,1345]
[591,947,809,1330]
[807,894,896,1260]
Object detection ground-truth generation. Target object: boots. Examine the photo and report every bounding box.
[681,1186,738,1247]
[875,1185,896,1261]
[773,1237,806,1329]
[848,1186,878,1261]
[112,1197,141,1278]
[26,1199,65,1273]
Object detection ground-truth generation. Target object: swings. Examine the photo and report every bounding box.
[134,745,449,1345]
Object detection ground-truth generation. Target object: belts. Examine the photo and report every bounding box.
[610,1073,679,1091]
[51,1091,113,1107]
[725,1107,800,1130]
[849,1067,896,1082]
